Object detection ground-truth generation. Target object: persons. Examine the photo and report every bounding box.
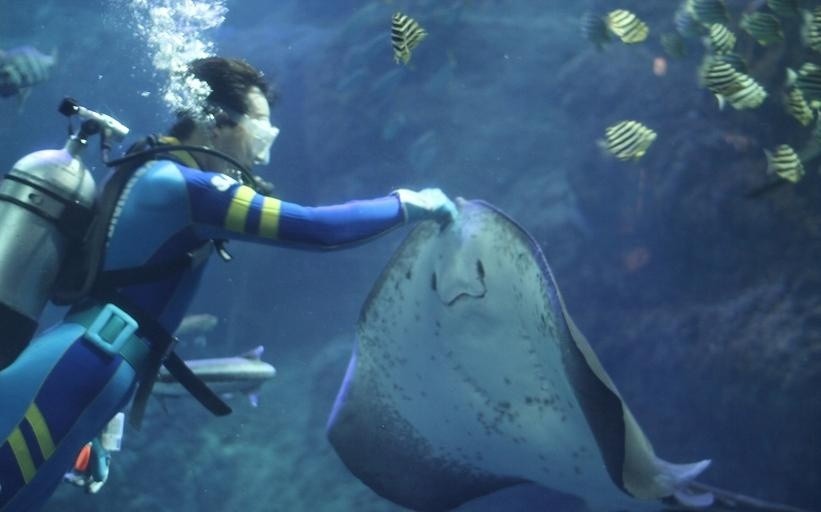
[0,59,457,504]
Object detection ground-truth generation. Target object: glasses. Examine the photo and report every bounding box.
[206,100,278,162]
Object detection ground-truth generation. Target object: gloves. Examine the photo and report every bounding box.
[64,437,110,493]
[390,188,460,232]
